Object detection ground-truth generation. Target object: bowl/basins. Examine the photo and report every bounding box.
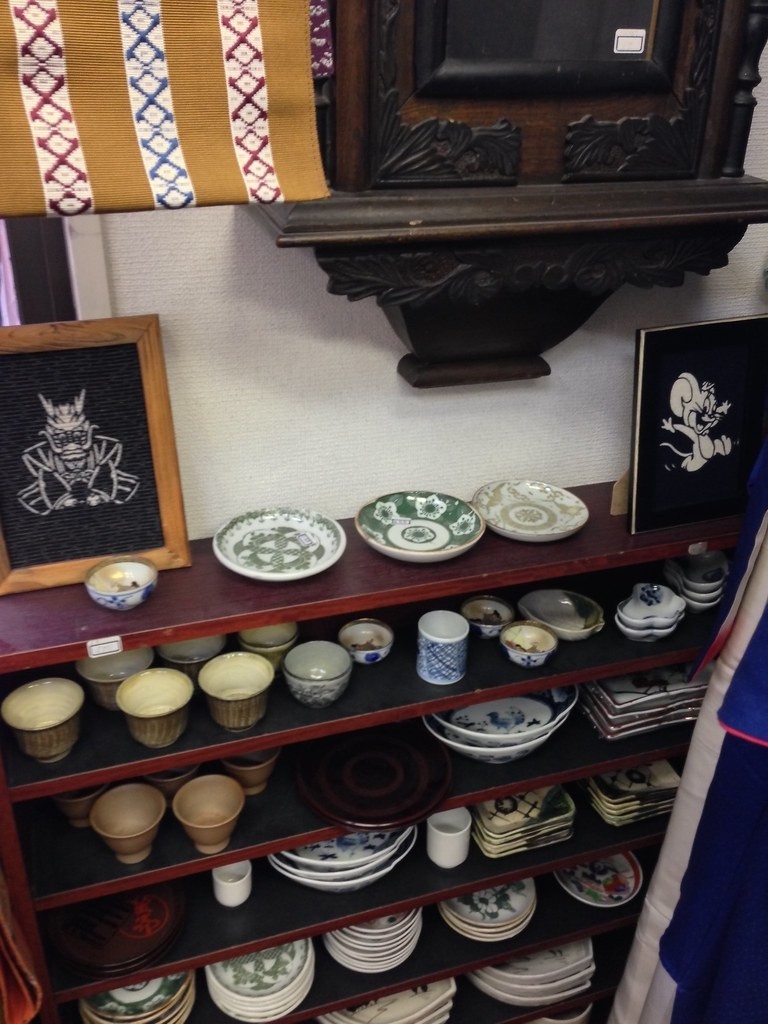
[422,681,579,765]
[266,826,418,893]
[339,619,394,665]
[500,621,558,668]
[85,557,158,611]
[464,600,514,637]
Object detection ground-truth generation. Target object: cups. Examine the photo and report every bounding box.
[144,763,200,807]
[77,649,153,710]
[172,775,246,855]
[2,678,84,763]
[157,634,228,690]
[212,859,252,908]
[117,668,194,748]
[427,806,472,870]
[92,784,167,865]
[198,651,276,732]
[283,641,352,709]
[222,746,281,796]
[417,611,470,686]
[57,784,106,828]
[239,623,300,680]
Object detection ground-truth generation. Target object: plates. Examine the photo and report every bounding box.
[295,724,458,832]
[582,759,683,827]
[553,852,644,908]
[439,877,538,942]
[517,590,606,641]
[615,551,729,642]
[318,978,456,1024]
[323,907,424,973]
[354,491,485,562]
[213,505,346,583]
[205,937,316,1020]
[578,659,718,741]
[467,937,596,1007]
[79,972,196,1024]
[472,480,589,542]
[59,888,185,974]
[471,785,575,858]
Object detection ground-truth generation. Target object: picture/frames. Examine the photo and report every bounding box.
[1,313,192,596]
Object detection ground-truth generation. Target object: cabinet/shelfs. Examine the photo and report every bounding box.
[0,492,736,1022]
